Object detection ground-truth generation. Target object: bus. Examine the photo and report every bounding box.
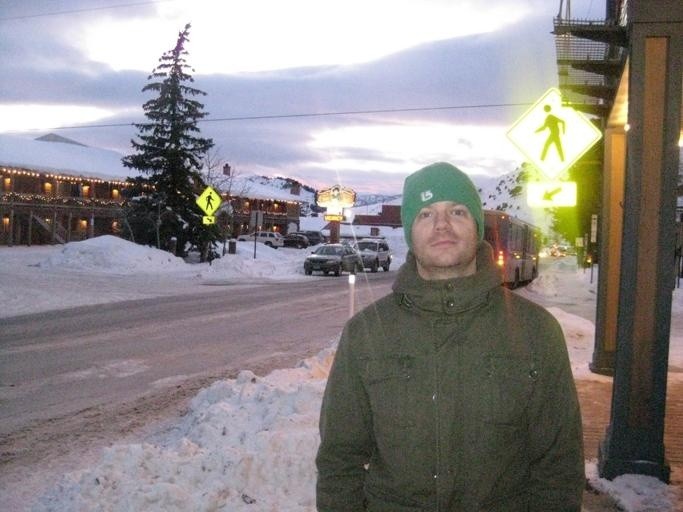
[479,209,547,292]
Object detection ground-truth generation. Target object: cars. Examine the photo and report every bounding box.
[304,242,363,276]
[540,239,576,262]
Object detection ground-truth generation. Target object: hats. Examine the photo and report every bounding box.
[402,162,484,248]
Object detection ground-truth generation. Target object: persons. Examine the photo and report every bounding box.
[313,160,587,512]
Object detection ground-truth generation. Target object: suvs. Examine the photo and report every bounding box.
[351,237,392,273]
[290,229,322,246]
[237,231,284,250]
[280,233,311,249]
